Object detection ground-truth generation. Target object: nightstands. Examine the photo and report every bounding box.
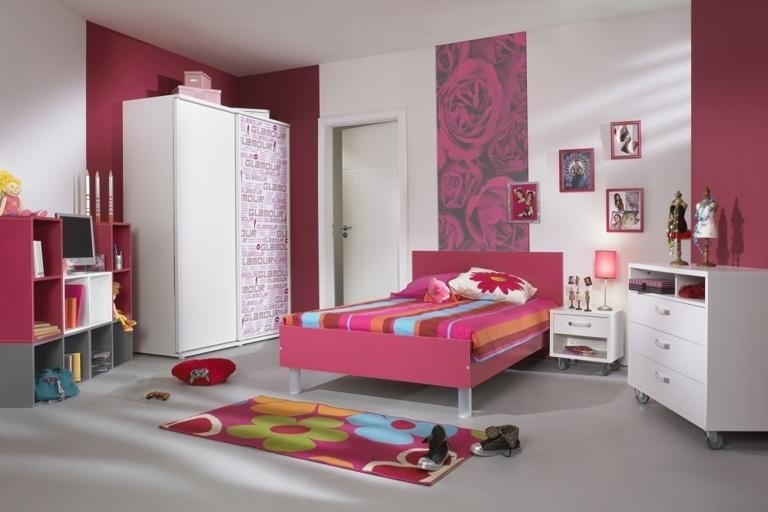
[550,306,628,376]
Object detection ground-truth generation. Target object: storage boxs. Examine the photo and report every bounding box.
[170,85,222,104]
[233,108,271,119]
[184,71,212,89]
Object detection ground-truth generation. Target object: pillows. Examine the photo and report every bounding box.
[390,272,458,299]
[448,266,538,304]
[171,358,236,386]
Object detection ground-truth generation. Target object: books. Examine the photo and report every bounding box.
[65,284,84,328]
[64,297,77,330]
[67,352,81,380]
[564,344,597,355]
[32,240,45,279]
[64,353,73,373]
[91,350,111,372]
[34,320,63,339]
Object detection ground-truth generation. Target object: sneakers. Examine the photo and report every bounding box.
[416,423,450,471]
[468,424,522,457]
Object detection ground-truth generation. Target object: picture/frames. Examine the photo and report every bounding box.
[507,182,541,223]
[611,120,641,159]
[559,149,594,192]
[606,188,644,233]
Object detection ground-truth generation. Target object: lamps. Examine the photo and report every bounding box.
[595,251,616,310]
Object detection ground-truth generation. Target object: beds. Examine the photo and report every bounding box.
[280,250,564,417]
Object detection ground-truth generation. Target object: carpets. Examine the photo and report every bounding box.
[159,395,490,487]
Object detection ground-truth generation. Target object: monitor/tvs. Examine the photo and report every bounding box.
[55,213,96,266]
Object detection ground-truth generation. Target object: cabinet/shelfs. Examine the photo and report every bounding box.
[626,263,768,451]
[122,93,292,359]
[1,216,133,408]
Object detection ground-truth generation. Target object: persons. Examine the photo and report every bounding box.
[611,213,622,228]
[668,191,689,233]
[515,189,526,204]
[0,169,49,219]
[626,191,640,211]
[693,188,720,238]
[585,277,592,286]
[622,211,640,231]
[518,206,534,218]
[112,281,137,333]
[614,194,624,211]
[569,276,574,284]
[524,191,534,206]
[571,158,583,177]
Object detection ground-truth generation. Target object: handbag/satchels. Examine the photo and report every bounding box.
[35,365,80,402]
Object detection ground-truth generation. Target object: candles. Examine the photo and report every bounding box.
[83,169,116,198]
